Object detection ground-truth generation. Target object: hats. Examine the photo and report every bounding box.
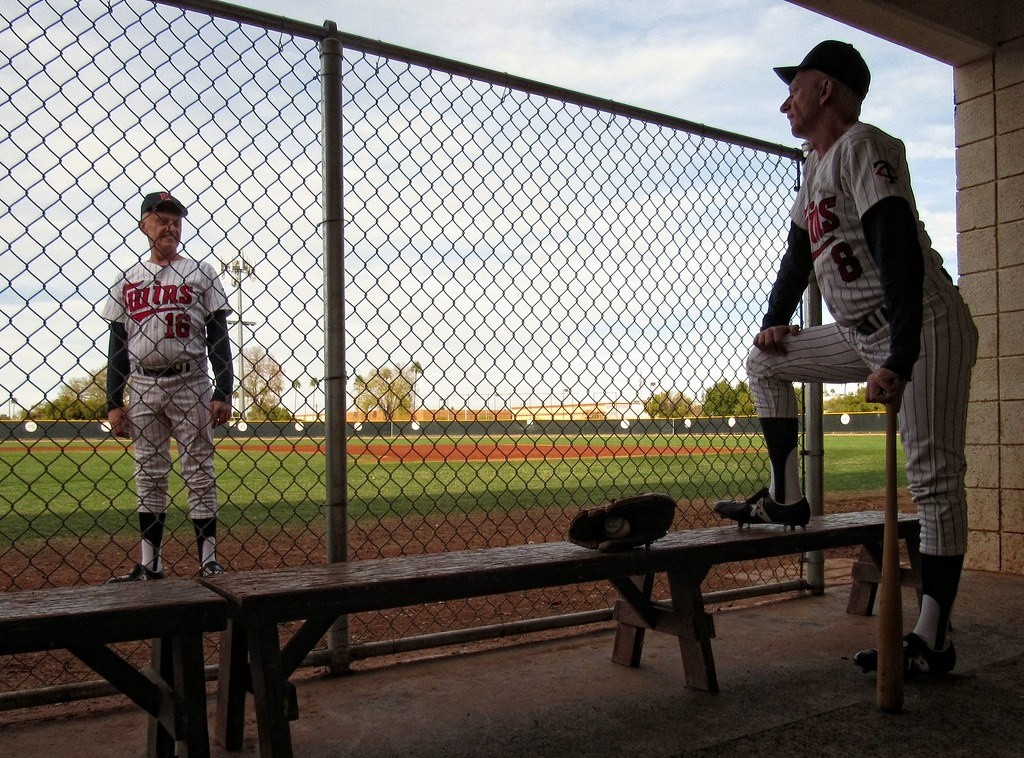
[773,40,871,97]
[141,192,188,217]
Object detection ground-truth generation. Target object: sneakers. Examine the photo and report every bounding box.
[198,561,228,577]
[106,564,164,584]
[853,633,956,676]
[714,487,811,531]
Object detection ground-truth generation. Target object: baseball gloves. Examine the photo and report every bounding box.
[568,491,678,551]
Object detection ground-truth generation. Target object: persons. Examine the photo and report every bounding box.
[714,40,979,676]
[100,191,234,583]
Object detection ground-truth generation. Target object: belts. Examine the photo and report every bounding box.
[136,362,189,377]
[855,268,952,336]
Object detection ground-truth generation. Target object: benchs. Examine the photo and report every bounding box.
[0,508,923,758]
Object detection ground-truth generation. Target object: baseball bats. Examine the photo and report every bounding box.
[872,390,910,713]
